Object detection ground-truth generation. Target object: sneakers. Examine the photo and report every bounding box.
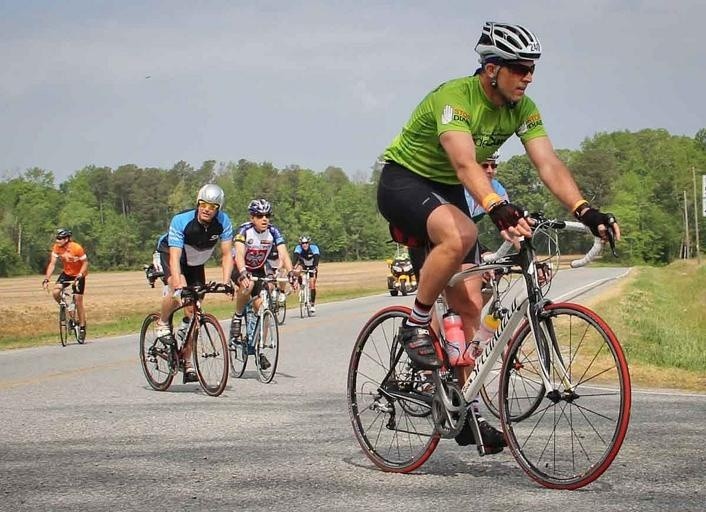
[60,307,65,320]
[155,293,315,382]
[79,329,86,339]
[455,414,507,448]
[398,317,442,369]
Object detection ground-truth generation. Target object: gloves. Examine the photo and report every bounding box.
[488,200,530,232]
[580,208,616,239]
[287,271,299,286]
[237,270,252,282]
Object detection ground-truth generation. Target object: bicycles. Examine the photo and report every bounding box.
[292,269,312,319]
[228,273,292,385]
[46,279,86,347]
[139,280,234,397]
[346,212,632,490]
[271,286,286,325]
[395,258,555,424]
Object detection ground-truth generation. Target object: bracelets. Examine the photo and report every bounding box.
[237,266,246,274]
[571,198,589,220]
[481,192,503,212]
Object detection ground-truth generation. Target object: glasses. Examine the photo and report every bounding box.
[496,61,535,76]
[198,200,219,210]
[482,162,497,169]
[255,214,271,218]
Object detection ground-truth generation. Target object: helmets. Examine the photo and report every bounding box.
[196,184,224,212]
[57,227,70,238]
[247,199,273,216]
[299,235,311,243]
[475,22,541,61]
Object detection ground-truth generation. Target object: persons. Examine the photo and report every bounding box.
[153,180,233,385]
[377,18,622,454]
[232,197,294,371]
[459,146,508,307]
[400,245,410,258]
[263,241,286,304]
[42,227,90,344]
[289,231,322,316]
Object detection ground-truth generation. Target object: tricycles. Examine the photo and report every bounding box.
[386,246,417,297]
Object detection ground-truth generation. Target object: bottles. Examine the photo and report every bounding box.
[175,315,189,342]
[442,308,500,366]
